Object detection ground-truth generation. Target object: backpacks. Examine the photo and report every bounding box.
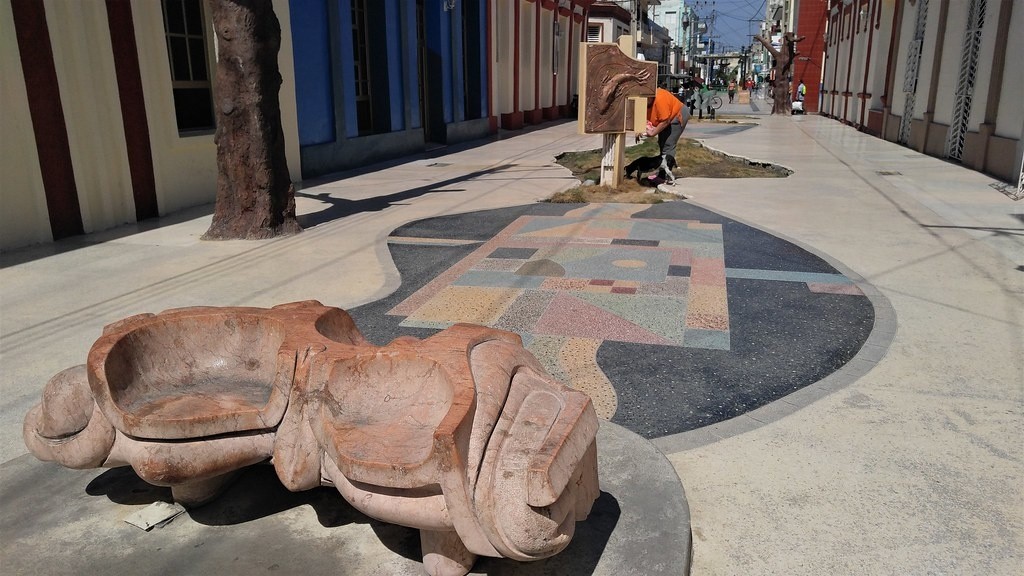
[799,85,806,95]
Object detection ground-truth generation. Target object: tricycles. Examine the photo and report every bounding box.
[657,74,723,109]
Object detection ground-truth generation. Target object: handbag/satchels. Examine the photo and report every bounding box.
[792,97,803,110]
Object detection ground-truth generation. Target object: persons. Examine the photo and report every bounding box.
[727,78,735,103]
[746,78,754,97]
[635,87,690,184]
[791,80,807,115]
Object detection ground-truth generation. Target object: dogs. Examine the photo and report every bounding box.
[623,154,678,187]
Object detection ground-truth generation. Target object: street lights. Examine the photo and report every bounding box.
[741,45,752,90]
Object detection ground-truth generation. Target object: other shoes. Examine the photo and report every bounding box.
[648,175,668,184]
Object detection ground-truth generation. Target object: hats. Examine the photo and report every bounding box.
[730,79,734,83]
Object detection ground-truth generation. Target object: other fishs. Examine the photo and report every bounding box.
[598,68,652,115]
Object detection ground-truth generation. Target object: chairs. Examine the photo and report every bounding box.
[19,298,601,576]
